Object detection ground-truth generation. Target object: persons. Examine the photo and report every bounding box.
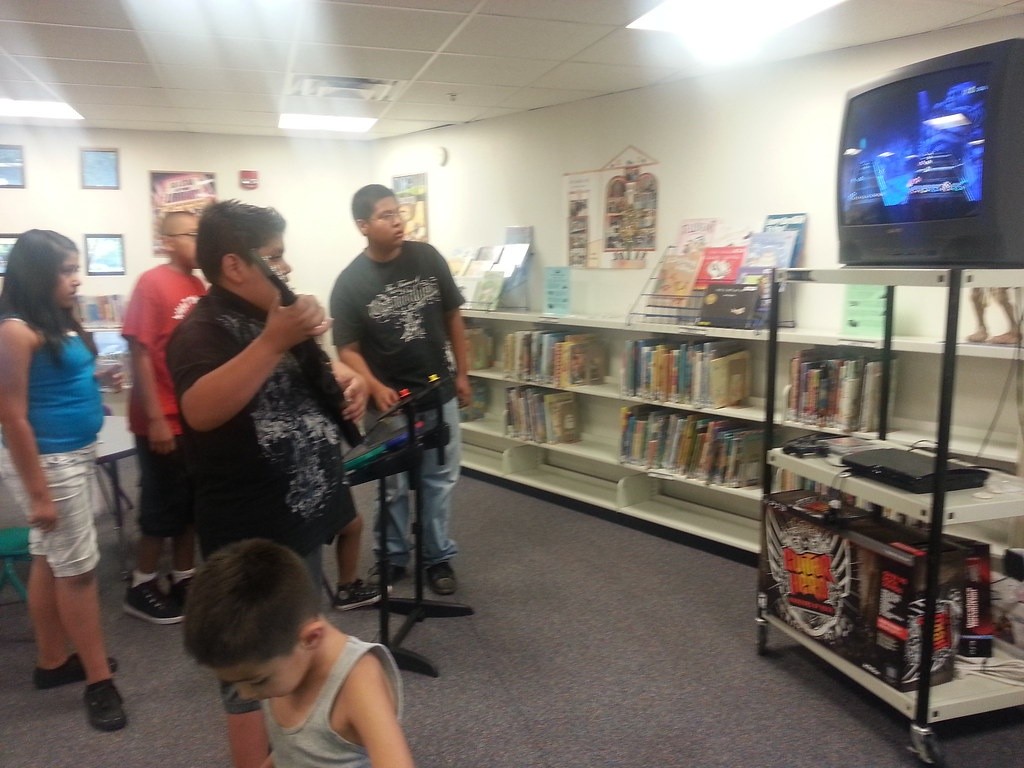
[0,229,127,733]
[180,539,413,768]
[327,182,474,594]
[165,200,395,612]
[113,210,208,625]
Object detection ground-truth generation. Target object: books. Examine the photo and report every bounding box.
[456,377,485,421]
[618,403,778,489]
[787,343,900,437]
[70,292,131,325]
[500,328,609,387]
[444,240,530,310]
[619,336,756,409]
[772,466,935,538]
[502,382,582,445]
[445,323,496,370]
[648,211,805,329]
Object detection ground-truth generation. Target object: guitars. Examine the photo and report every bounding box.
[245,240,365,444]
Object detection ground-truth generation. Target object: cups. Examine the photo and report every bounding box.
[96,353,118,393]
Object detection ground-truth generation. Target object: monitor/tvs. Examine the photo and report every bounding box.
[835,38,1024,270]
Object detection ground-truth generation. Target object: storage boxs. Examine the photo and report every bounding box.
[761,487,992,693]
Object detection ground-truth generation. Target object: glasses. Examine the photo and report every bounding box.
[168,230,202,241]
[367,208,408,230]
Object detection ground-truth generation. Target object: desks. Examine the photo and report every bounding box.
[91,413,145,583]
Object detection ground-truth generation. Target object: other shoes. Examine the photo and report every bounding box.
[365,563,406,587]
[84,677,128,731]
[425,558,458,595]
[35,652,120,689]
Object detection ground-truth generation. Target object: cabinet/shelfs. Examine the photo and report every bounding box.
[444,308,1024,556]
[753,263,1024,768]
[82,319,133,394]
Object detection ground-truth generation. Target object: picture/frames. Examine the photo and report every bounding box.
[85,233,126,275]
[78,147,119,190]
[0,233,20,276]
[0,143,25,188]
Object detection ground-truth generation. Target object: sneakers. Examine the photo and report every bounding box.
[333,575,392,612]
[121,578,190,624]
[167,571,196,606]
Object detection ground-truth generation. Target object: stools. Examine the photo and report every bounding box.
[0,526,29,609]
